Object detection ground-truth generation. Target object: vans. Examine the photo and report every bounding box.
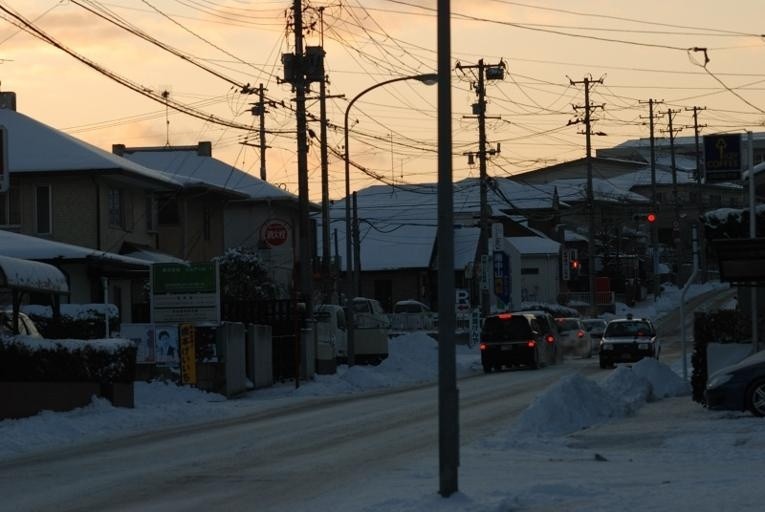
[394,301,431,330]
[312,297,388,374]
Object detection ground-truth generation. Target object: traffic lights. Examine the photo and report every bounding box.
[632,213,656,223]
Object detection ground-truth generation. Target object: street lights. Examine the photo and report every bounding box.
[345,74,438,368]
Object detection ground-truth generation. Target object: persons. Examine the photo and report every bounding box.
[158,330,176,360]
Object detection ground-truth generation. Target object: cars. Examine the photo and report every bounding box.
[599,319,661,369]
[704,349,765,417]
[480,310,607,373]
